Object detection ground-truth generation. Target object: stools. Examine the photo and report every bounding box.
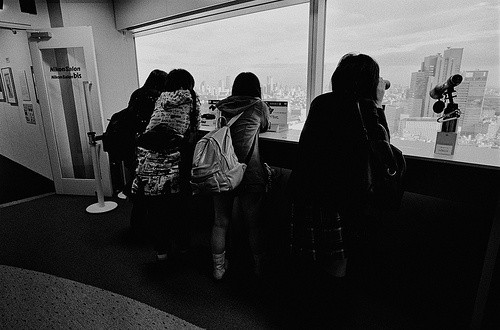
[288,198,345,264]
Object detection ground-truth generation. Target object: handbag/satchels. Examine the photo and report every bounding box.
[378,140,407,211]
[134,123,187,154]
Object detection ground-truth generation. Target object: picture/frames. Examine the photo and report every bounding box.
[0,66,40,124]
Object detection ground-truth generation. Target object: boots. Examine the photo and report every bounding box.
[213,250,228,280]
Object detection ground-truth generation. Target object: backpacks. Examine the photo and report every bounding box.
[189,112,257,197]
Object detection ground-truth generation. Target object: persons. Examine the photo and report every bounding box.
[130,69,200,261]
[124,69,169,250]
[210,72,271,280]
[289,53,405,330]
